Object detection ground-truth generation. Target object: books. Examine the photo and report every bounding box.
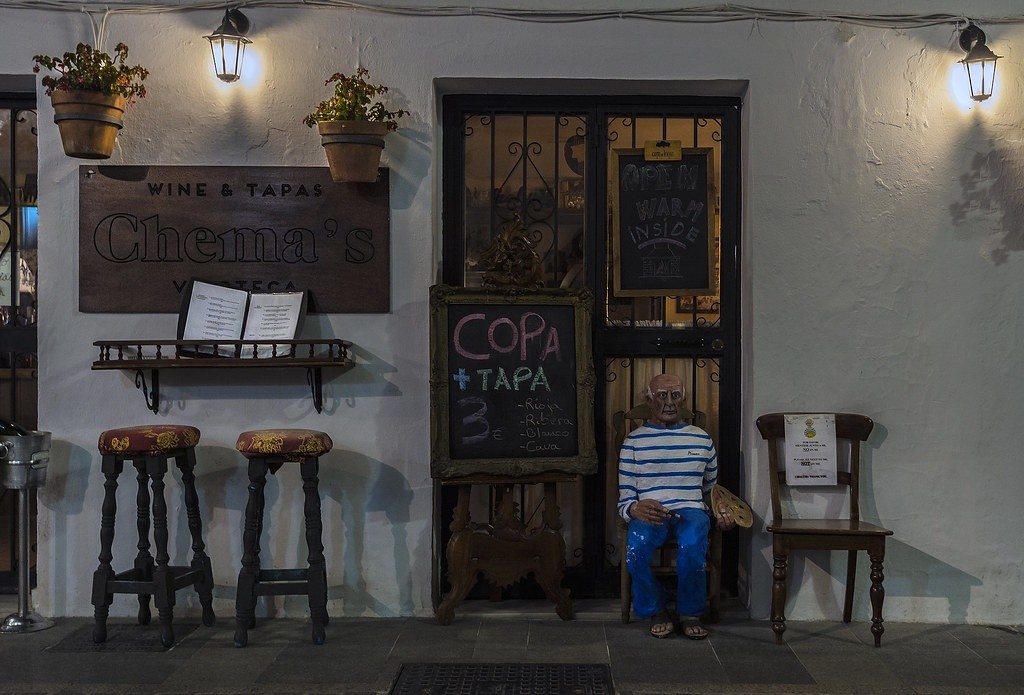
[175,274,308,360]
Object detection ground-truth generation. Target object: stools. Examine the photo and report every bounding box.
[86,424,217,648]
[226,423,332,646]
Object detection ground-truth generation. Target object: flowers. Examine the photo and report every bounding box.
[28,41,152,103]
[301,65,412,134]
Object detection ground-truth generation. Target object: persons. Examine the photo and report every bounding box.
[615,373,737,639]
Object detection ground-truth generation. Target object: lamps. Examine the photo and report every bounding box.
[956,26,1005,102]
[202,6,253,85]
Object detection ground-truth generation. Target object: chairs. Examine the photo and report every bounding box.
[755,412,893,647]
[614,400,723,626]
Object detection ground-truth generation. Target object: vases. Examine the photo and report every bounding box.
[318,119,388,184]
[49,91,126,159]
